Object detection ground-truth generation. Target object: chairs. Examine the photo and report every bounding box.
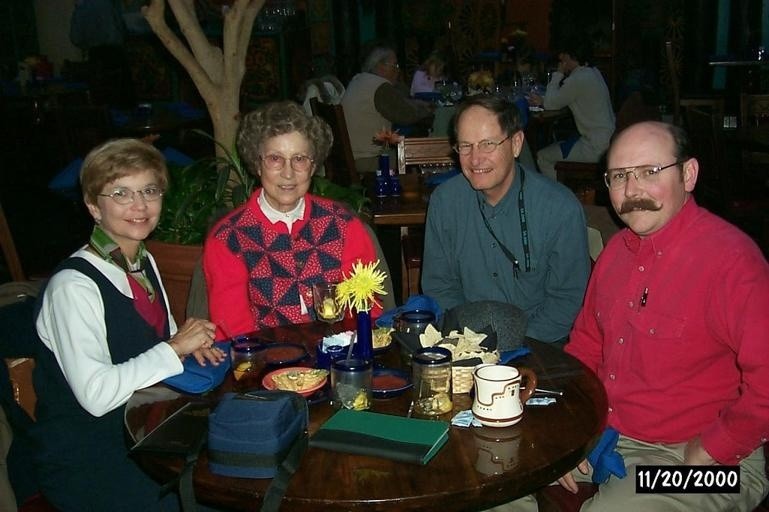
[1,38,213,287]
[306,38,769,309]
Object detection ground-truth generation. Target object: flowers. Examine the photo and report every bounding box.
[334,257,390,316]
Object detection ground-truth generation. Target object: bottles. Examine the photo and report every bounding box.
[376,171,403,198]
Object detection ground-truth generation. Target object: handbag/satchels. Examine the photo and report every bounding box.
[207,388,309,479]
[166,340,231,395]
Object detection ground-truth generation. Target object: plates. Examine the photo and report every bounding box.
[262,368,329,396]
[374,340,394,356]
[370,369,414,401]
[263,340,306,373]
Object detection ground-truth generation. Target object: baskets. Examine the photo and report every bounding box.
[451,351,499,394]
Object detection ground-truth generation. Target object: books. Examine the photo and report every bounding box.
[307,408,452,466]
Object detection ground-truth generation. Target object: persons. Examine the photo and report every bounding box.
[203,99,384,343]
[29,137,228,512]
[482,120,769,512]
[340,32,617,184]
[420,96,592,346]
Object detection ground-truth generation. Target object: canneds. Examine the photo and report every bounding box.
[403,312,436,337]
[332,359,372,409]
[232,340,266,391]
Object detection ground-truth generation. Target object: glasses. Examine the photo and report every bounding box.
[603,162,679,189]
[260,155,316,173]
[100,185,163,205]
[453,135,510,155]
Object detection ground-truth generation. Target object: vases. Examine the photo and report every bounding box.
[353,310,375,365]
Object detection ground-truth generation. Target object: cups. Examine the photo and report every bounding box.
[471,363,538,426]
[470,425,521,476]
[316,338,363,373]
[329,356,375,413]
[400,310,437,373]
[227,338,262,392]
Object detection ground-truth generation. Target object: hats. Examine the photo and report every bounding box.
[443,300,527,353]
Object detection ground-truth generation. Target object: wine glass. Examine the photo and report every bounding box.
[493,66,553,98]
[311,280,351,339]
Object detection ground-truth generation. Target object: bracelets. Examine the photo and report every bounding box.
[167,339,184,358]
